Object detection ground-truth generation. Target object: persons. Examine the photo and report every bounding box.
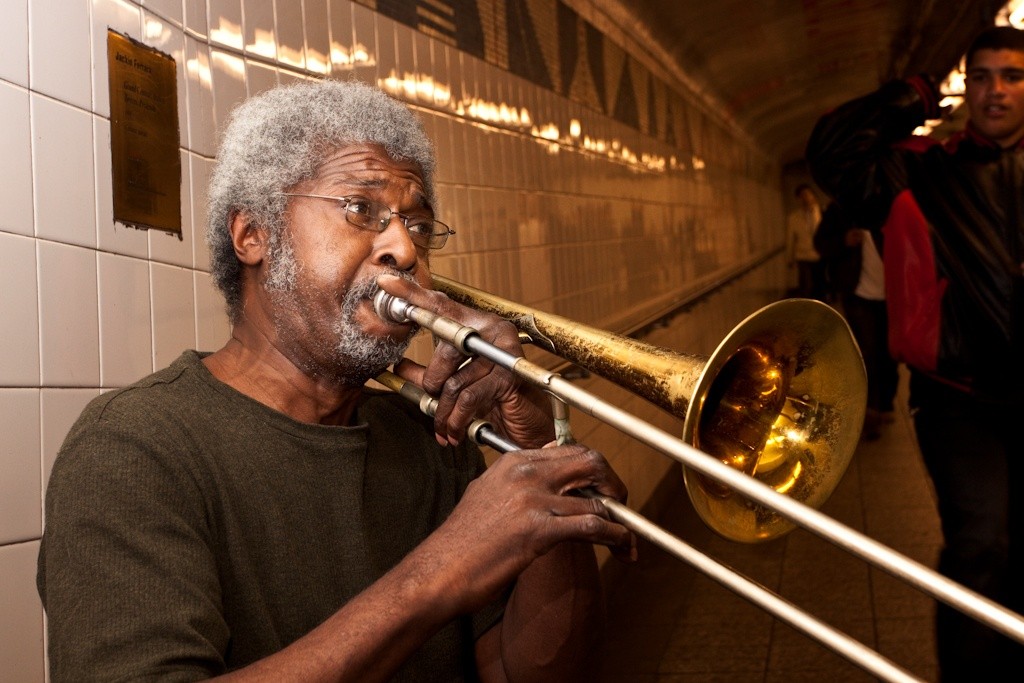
[32,80,626,683]
[788,162,898,441]
[803,24,1024,683]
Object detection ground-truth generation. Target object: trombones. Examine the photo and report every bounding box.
[372,272,1024,683]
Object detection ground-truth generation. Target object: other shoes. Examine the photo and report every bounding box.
[862,409,883,443]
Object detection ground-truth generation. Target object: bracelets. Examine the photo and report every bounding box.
[911,75,936,117]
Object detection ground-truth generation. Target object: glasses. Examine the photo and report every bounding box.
[287,194,456,250]
